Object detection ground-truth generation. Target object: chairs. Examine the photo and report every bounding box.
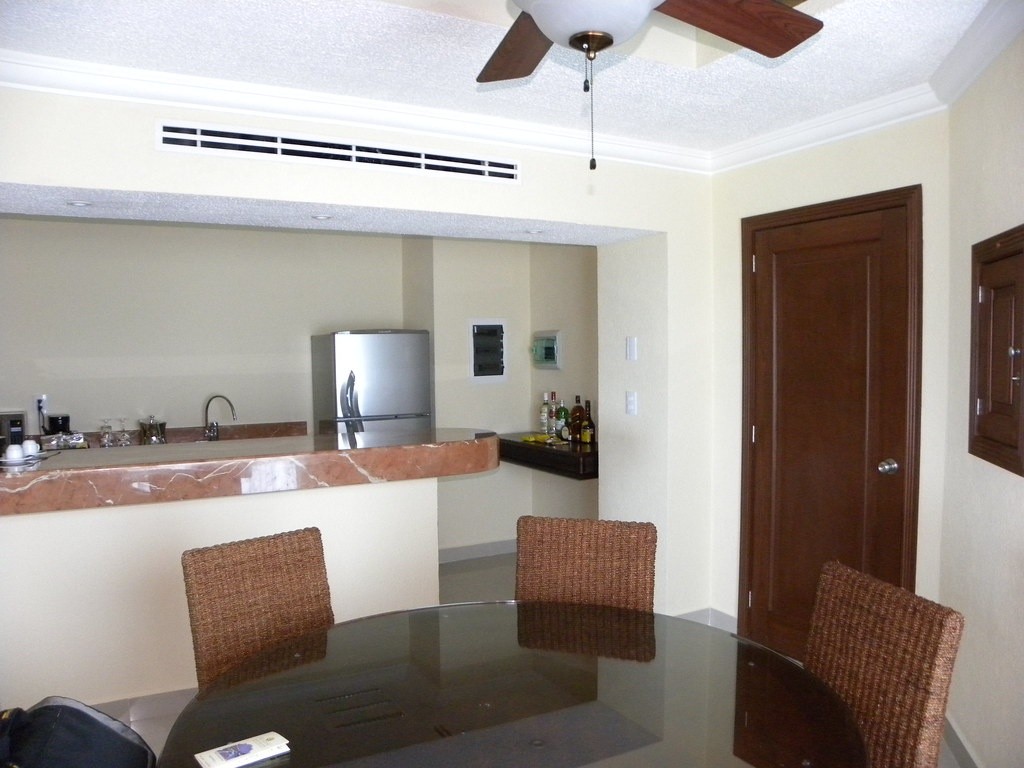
[515,515,655,611]
[806,561,963,767]
[181,526,336,687]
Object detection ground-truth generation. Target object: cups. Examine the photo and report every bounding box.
[22,439,40,456]
[6,444,23,459]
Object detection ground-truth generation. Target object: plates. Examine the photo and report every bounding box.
[0,456,32,462]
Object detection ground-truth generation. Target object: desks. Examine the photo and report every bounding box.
[158,602,871,768]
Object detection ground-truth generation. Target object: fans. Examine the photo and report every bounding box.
[477,0,824,81]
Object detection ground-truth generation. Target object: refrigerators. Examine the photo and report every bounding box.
[312,324,433,437]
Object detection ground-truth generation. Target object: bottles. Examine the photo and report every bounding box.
[560,414,572,441]
[555,399,569,441]
[580,399,596,443]
[571,394,585,441]
[549,390,558,435]
[540,392,549,434]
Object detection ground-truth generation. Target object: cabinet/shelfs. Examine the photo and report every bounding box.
[498,433,598,479]
[967,222,1024,478]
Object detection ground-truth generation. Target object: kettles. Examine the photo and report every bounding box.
[42,414,71,436]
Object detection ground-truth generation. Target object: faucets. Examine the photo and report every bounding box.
[202,394,239,439]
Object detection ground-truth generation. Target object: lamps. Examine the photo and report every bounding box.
[514,1,666,170]
